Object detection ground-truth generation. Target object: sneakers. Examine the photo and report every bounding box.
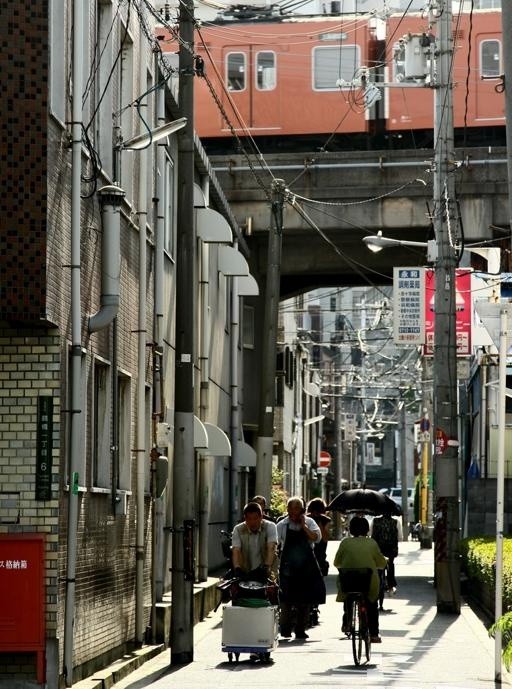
[370,632,382,643]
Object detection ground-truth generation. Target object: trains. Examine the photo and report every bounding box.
[148,6,506,142]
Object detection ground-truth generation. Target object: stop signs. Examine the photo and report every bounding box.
[319,452,332,467]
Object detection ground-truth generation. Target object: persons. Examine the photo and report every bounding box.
[227,494,276,522]
[276,496,322,640]
[372,513,398,593]
[308,498,331,580]
[231,502,279,663]
[335,516,389,643]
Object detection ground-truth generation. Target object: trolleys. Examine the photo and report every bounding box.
[219,571,284,666]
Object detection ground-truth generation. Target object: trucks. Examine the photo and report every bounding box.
[378,487,416,513]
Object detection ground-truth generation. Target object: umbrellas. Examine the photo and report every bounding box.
[325,484,401,517]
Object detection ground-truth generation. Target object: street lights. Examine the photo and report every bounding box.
[359,0,466,613]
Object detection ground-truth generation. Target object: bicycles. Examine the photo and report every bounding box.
[338,566,376,665]
[377,560,393,611]
[220,529,234,561]
